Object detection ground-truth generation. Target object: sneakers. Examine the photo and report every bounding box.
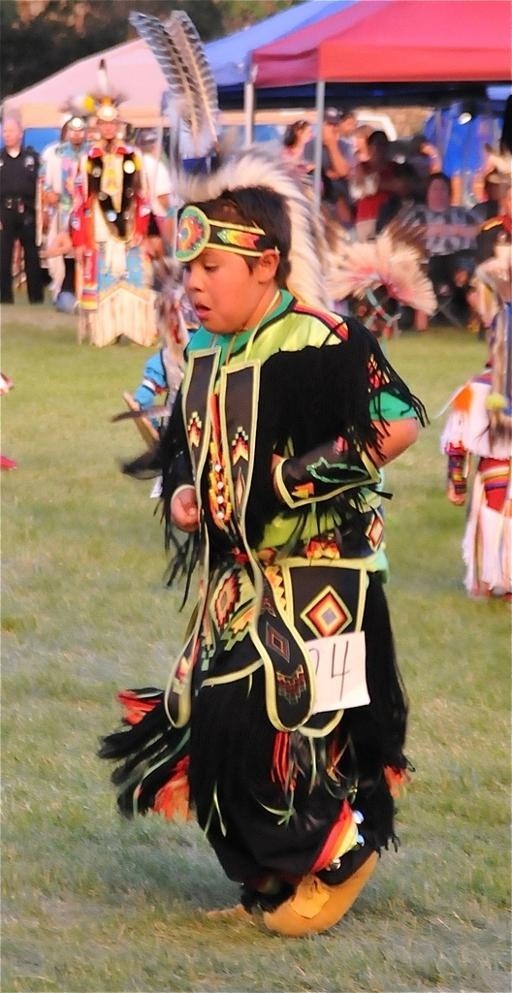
[263,849,379,937]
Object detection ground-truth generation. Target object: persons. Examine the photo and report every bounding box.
[98,189,422,938]
[275,108,512,600]
[122,270,200,501]
[0,91,175,345]
